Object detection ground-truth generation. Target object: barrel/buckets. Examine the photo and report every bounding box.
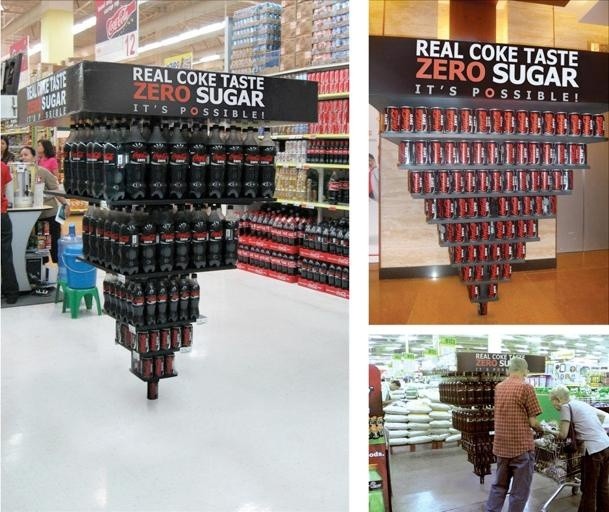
[56,223,84,282]
[62,244,97,289]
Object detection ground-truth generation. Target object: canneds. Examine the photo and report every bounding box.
[232,0,349,166]
[469,285,480,301]
[474,264,484,282]
[26,220,51,253]
[384,106,606,262]
[503,263,512,279]
[461,265,472,280]
[479,302,487,315]
[115,321,194,399]
[488,264,500,281]
[468,454,496,484]
[487,285,496,299]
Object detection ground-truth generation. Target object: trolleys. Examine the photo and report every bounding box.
[527,424,584,511]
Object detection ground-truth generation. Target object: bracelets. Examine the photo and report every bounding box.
[63,203,67,208]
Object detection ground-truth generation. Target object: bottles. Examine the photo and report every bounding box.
[326,168,349,204]
[99,271,201,326]
[82,201,240,274]
[64,124,277,199]
[306,141,349,164]
[240,209,348,293]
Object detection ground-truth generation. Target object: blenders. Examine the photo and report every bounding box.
[14,162,37,208]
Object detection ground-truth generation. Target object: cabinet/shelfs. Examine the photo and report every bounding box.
[224,62,348,300]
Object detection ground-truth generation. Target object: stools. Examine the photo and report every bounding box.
[55,281,101,318]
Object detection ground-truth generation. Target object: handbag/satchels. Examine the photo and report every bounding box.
[563,421,577,454]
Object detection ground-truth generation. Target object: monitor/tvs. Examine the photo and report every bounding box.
[1,53,22,95]
[525,354,546,373]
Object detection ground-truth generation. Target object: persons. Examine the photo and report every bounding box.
[19,146,71,263]
[1,161,20,304]
[35,138,59,179]
[381,379,401,401]
[484,356,545,511]
[0,135,15,165]
[544,384,609,511]
[368,153,378,202]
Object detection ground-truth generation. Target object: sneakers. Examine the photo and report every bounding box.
[7,297,17,304]
